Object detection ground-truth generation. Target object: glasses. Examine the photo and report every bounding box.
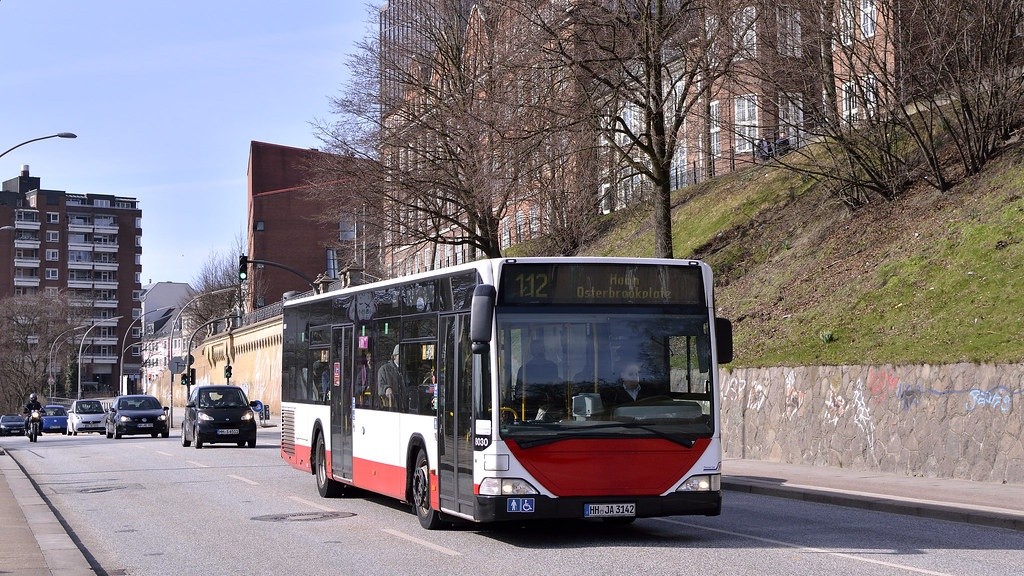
[626,372,639,377]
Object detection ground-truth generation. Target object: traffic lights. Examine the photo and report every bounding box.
[181,374,187,385]
[239,255,247,280]
[224,366,232,378]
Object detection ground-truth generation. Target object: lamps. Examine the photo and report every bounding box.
[254,221,265,231]
[254,259,265,269]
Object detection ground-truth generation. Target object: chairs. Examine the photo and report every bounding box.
[121,400,129,407]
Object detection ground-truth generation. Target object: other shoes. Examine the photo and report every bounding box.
[26,432,29,435]
[38,433,42,436]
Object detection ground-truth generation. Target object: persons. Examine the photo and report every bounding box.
[614,361,658,404]
[378,344,399,399]
[755,133,774,161]
[23,394,44,436]
[774,132,790,157]
[515,340,559,413]
[355,350,372,395]
[313,361,330,396]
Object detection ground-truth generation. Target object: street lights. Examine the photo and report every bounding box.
[54,334,84,397]
[120,341,142,396]
[119,305,176,396]
[187,315,242,401]
[170,286,237,428]
[77,315,124,399]
[81,336,107,399]
[49,324,86,398]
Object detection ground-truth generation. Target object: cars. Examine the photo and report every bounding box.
[66,399,108,435]
[40,405,68,435]
[0,413,26,436]
[104,395,169,440]
[181,385,257,449]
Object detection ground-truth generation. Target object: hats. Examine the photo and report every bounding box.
[393,344,400,355]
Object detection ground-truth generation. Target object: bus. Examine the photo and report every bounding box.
[280,257,733,529]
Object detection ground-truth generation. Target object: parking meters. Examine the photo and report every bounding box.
[264,404,269,425]
[259,409,263,426]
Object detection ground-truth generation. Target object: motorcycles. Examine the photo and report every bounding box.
[22,405,45,442]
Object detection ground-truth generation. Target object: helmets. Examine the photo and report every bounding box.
[30,394,37,402]
